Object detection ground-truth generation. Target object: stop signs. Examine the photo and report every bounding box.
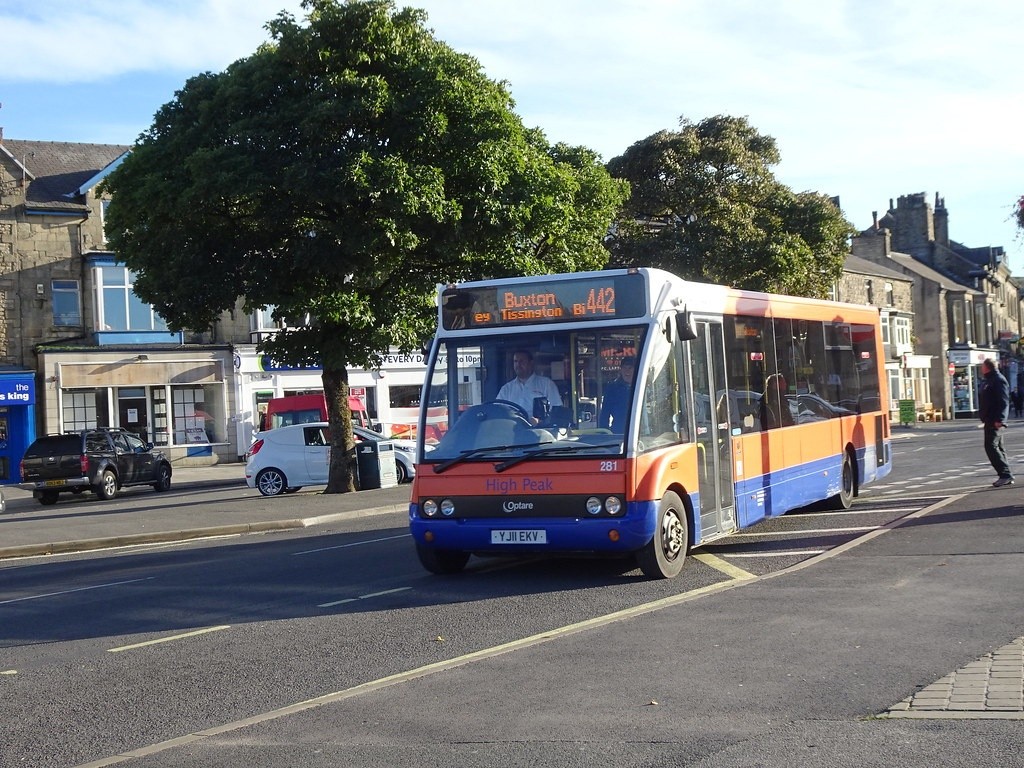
[949,363,956,377]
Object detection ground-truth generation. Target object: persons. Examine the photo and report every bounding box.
[979,357,1024,487]
[598,358,651,435]
[760,374,796,431]
[492,350,564,430]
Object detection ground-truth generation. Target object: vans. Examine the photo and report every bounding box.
[264,394,375,449]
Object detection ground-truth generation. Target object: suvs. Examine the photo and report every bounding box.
[18,427,173,505]
[243,422,437,496]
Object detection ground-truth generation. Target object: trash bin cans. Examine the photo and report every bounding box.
[355,440,398,489]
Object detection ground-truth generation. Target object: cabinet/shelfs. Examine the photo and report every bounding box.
[953,381,970,398]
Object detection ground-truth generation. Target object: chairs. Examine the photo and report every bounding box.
[307,432,321,446]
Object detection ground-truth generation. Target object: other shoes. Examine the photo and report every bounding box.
[993,477,1014,487]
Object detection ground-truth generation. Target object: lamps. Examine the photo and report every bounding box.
[134,355,148,360]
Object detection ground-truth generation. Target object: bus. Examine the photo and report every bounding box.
[407,266,894,580]
